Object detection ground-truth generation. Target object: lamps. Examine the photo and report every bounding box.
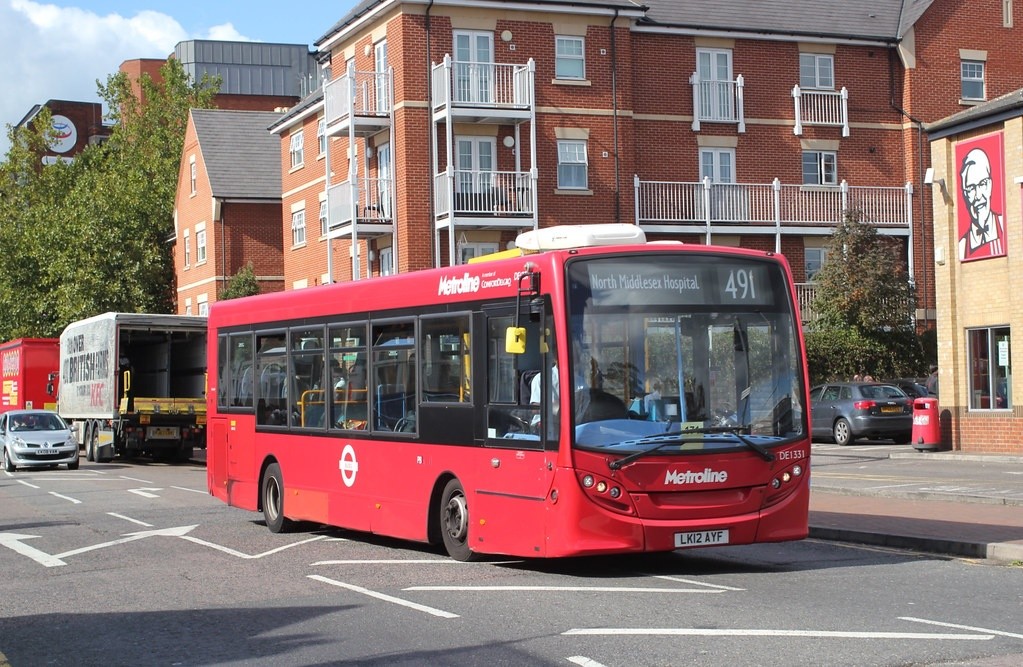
[366,147,374,158]
[369,250,376,262]
[364,45,371,57]
[1014,176,1023,184]
[502,136,515,148]
[500,30,512,42]
[923,168,944,186]
[506,241,517,250]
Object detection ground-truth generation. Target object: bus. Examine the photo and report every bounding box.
[206,222,813,565]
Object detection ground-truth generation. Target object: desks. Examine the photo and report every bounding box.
[506,187,529,216]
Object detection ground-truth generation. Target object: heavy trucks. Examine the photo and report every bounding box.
[45,312,209,461]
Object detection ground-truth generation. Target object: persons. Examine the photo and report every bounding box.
[854,375,874,383]
[926,366,939,399]
[11,417,27,431]
[34,418,55,430]
[529,345,591,435]
[246,342,457,432]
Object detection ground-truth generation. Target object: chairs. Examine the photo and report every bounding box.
[242,363,287,406]
[319,361,415,401]
[364,190,387,224]
[295,340,318,362]
[491,187,512,215]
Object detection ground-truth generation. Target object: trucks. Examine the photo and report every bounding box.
[0,337,62,415]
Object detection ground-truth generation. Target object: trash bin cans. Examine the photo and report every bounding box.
[912,398,942,453]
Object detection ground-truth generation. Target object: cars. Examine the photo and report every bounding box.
[793,380,914,447]
[0,408,79,473]
[880,378,932,399]
